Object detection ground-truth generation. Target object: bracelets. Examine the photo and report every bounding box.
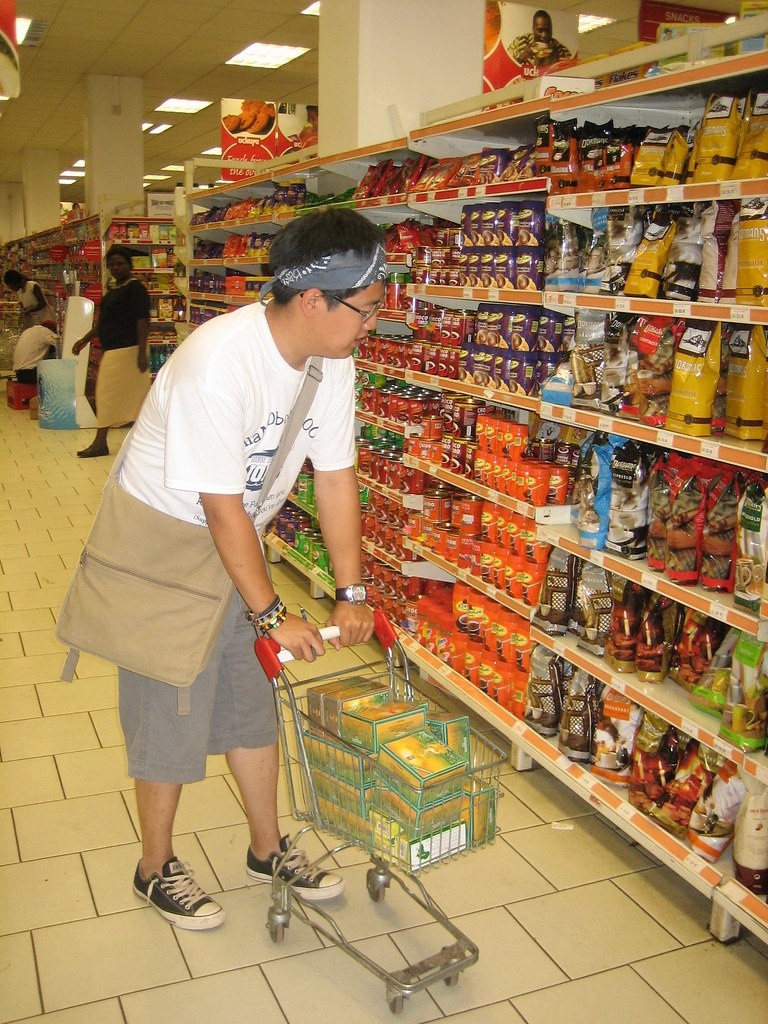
[242,593,287,634]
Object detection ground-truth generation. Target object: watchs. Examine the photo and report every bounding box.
[335,583,368,606]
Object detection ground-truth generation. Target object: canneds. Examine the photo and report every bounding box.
[265,151,579,720]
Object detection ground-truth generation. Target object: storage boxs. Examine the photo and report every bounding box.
[145,192,174,218]
[426,709,471,771]
[316,793,374,850]
[322,681,393,740]
[372,777,463,839]
[606,41,657,87]
[734,0,768,55]
[578,53,610,90]
[5,381,38,410]
[308,761,375,819]
[368,808,405,842]
[303,730,375,787]
[461,775,496,850]
[372,832,397,864]
[338,699,427,754]
[655,22,725,66]
[394,818,468,873]
[374,728,466,809]
[104,223,178,290]
[306,675,370,727]
[156,298,173,318]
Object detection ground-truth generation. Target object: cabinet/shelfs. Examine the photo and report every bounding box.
[0,193,181,378]
[183,49,767,948]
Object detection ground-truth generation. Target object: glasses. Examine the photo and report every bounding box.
[300,288,387,323]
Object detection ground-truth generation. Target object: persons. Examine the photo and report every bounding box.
[299,104,318,143]
[109,206,389,930]
[4,269,52,327]
[509,10,571,67]
[14,320,62,386]
[71,246,150,457]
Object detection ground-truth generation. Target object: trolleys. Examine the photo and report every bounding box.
[254,609,507,1014]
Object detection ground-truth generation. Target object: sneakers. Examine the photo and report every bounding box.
[247,836,343,899]
[132,856,224,929]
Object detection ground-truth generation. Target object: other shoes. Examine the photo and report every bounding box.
[77,442,109,458]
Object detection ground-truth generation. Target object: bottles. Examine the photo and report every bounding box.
[148,344,177,373]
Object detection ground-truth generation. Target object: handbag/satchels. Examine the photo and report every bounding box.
[54,485,237,686]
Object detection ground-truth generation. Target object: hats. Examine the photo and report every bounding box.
[41,318,58,330]
